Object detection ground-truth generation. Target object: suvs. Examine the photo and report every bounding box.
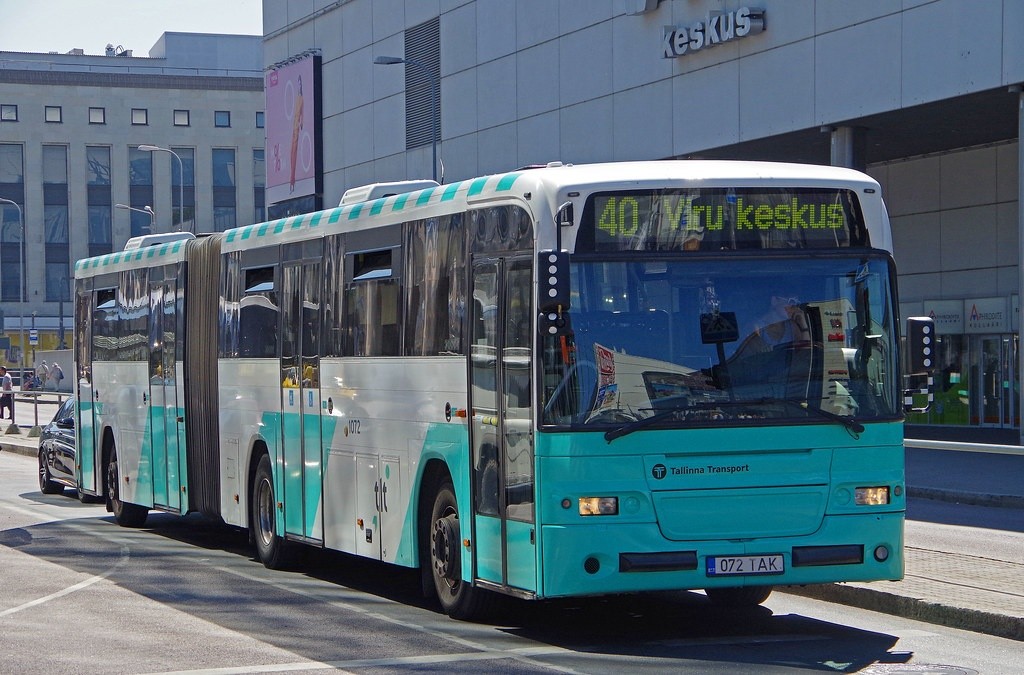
[686,309,891,420]
[468,352,534,506]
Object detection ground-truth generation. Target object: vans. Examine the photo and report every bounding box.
[585,341,723,421]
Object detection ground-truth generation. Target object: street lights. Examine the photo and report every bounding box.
[140,148,185,230]
[115,204,157,233]
[1,198,25,391]
[650,310,674,363]
[372,57,438,183]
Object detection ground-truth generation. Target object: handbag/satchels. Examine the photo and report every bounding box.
[0,396,10,407]
[59,370,63,379]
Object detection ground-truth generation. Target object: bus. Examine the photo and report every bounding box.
[72,156,939,611]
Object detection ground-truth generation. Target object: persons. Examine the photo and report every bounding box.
[0,366,13,419]
[289,75,304,194]
[742,281,814,410]
[25,360,64,392]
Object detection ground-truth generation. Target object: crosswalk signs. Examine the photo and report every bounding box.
[698,311,740,342]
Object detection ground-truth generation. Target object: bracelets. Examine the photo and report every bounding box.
[799,327,810,333]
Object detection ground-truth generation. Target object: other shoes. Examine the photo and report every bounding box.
[8,417,12,419]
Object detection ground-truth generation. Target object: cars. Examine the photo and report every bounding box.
[38,394,104,503]
[539,360,618,427]
